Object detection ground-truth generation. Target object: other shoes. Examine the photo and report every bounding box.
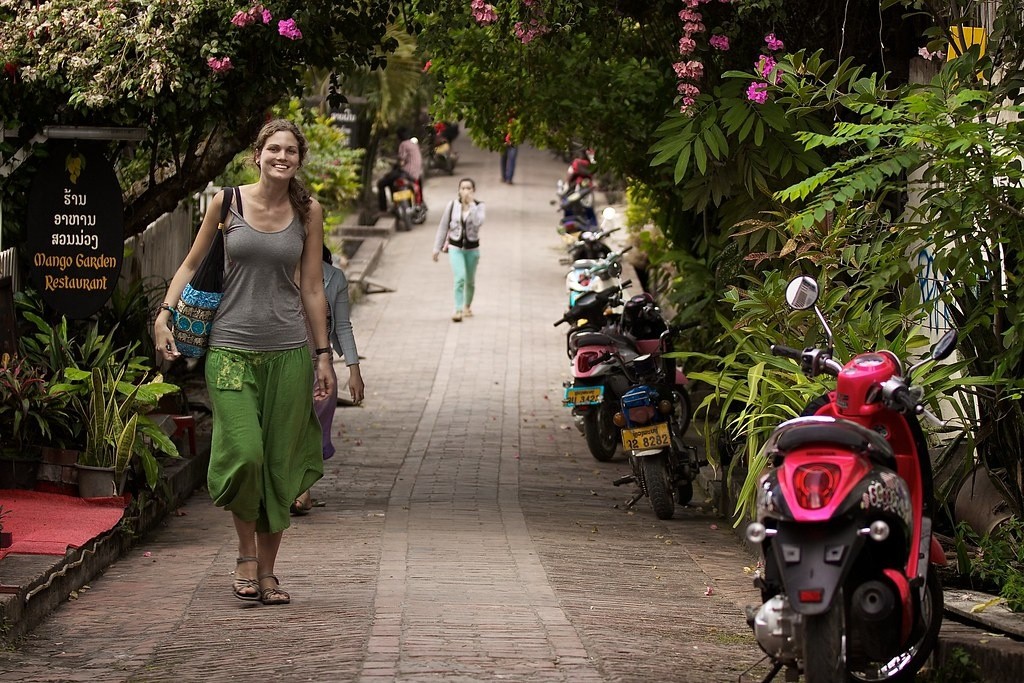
[452,310,462,322]
[463,308,472,317]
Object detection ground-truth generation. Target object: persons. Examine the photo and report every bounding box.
[567,156,594,190]
[432,178,485,322]
[430,116,447,144]
[370,126,421,219]
[154,120,335,603]
[498,103,521,187]
[292,234,366,516]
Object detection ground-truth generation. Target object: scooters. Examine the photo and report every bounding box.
[735,271,960,683]
[549,150,694,462]
[388,162,429,232]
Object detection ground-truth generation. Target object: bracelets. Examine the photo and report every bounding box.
[158,302,175,315]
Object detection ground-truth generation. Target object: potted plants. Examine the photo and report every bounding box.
[0,291,182,500]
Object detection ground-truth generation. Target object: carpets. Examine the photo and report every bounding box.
[0,489,132,561]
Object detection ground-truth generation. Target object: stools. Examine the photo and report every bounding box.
[164,413,197,456]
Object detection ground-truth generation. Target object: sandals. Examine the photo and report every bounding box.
[259,573,290,605]
[232,556,261,601]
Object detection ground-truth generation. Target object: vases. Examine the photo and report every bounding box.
[950,463,1017,546]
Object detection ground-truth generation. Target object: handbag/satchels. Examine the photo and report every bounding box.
[441,227,450,253]
[166,186,234,360]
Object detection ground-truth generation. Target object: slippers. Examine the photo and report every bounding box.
[291,493,310,517]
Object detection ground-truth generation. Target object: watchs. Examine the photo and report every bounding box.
[317,345,334,356]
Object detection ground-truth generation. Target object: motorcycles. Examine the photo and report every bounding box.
[584,317,711,522]
